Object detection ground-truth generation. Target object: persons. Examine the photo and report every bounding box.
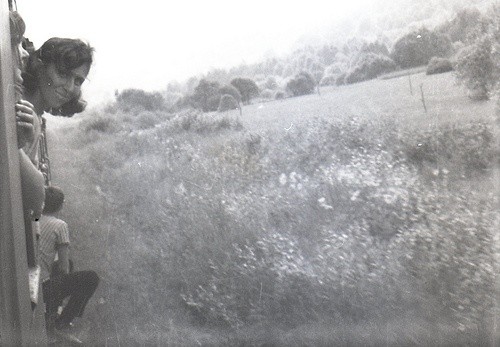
[21,37,95,114]
[38,186,100,343]
[9,9,45,306]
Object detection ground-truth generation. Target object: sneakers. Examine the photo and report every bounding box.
[51,319,82,343]
